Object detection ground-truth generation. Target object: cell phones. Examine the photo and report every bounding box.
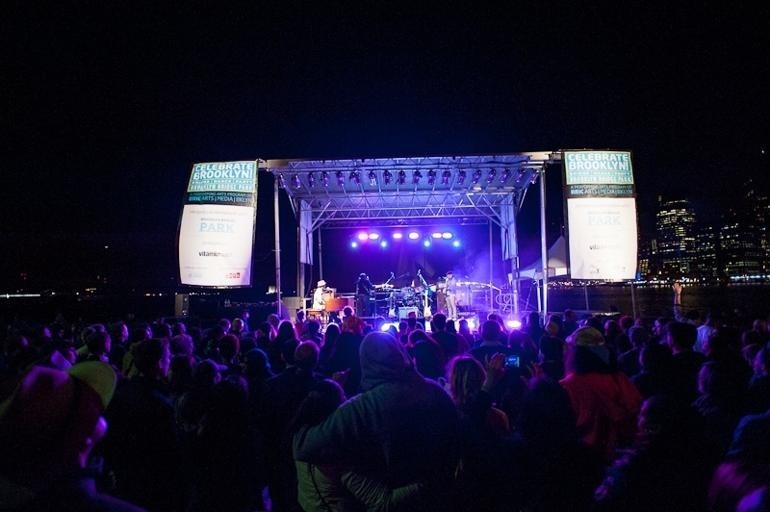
[502,355,521,370]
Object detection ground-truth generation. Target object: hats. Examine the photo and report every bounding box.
[244,348,271,368]
[482,322,500,341]
[81,324,105,342]
[232,318,245,332]
[565,326,605,347]
[295,340,314,359]
[318,280,326,287]
[18,361,119,469]
[220,335,241,354]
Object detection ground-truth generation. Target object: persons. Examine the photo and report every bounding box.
[1,269,768,512]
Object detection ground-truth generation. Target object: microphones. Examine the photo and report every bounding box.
[390,272,395,280]
[417,269,421,276]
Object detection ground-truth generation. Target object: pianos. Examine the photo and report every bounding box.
[326,296,355,313]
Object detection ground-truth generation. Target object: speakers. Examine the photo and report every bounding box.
[175,294,190,316]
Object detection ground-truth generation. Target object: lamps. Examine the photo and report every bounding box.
[273,169,528,190]
